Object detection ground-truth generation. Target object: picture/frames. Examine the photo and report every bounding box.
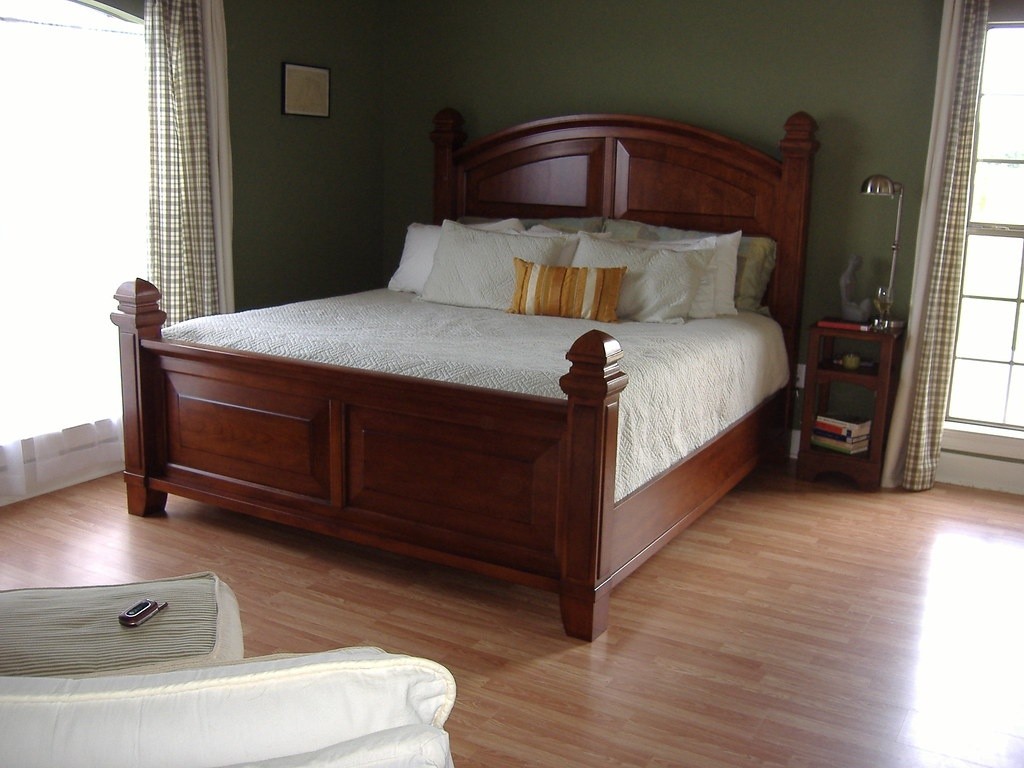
[280,63,332,118]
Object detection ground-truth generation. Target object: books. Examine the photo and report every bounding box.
[817,319,871,332]
[810,412,873,455]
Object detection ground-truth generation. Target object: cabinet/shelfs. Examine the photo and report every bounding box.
[796,316,901,493]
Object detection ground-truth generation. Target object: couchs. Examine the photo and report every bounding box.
[1,571,456,768]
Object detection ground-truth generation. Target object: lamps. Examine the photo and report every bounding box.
[859,174,907,329]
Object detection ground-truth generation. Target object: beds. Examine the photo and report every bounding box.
[109,107,820,643]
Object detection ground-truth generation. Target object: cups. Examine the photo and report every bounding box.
[843,352,861,371]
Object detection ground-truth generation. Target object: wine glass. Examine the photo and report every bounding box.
[870,287,898,334]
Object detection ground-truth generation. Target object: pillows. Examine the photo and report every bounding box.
[387,216,777,323]
